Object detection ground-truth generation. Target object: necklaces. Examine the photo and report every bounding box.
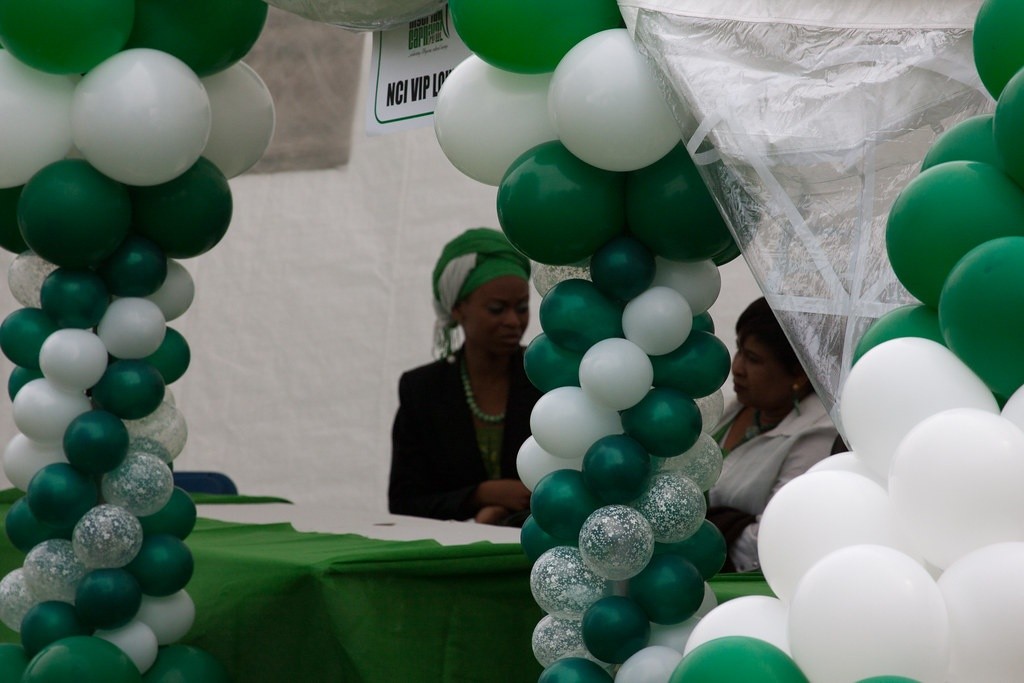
[460,350,506,424]
[731,408,778,451]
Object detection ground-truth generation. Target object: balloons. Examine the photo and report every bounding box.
[0,0,275,683]
[670,0,1024,683]
[548,28,681,172]
[497,140,627,266]
[627,139,762,267]
[433,55,560,186]
[524,222,731,459]
[516,431,726,683]
[449,0,627,74]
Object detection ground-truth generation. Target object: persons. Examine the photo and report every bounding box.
[388,228,547,529]
[703,296,849,574]
